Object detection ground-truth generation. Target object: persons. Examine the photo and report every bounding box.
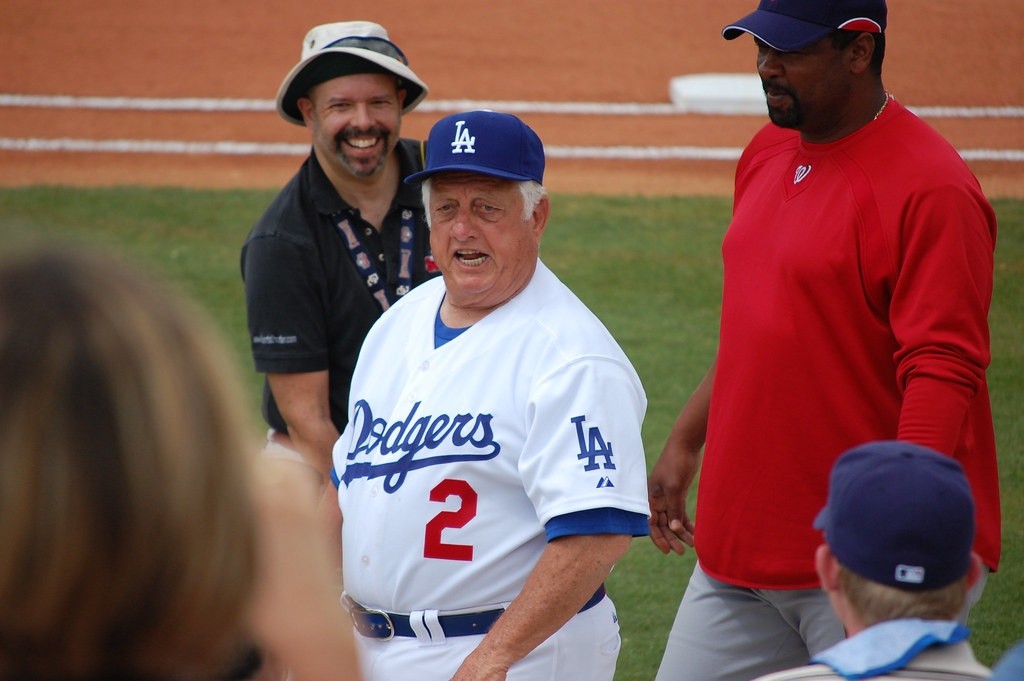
[751,443,1024,681]
[242,21,441,491]
[333,110,651,681]
[0,234,360,681]
[648,0,1003,681]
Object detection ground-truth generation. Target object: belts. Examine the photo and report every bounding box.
[342,580,605,642]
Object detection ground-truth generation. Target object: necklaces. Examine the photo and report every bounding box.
[873,93,889,120]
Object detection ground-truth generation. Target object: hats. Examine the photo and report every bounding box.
[722,0,887,51]
[276,21,428,127]
[812,442,976,590]
[404,110,545,188]
